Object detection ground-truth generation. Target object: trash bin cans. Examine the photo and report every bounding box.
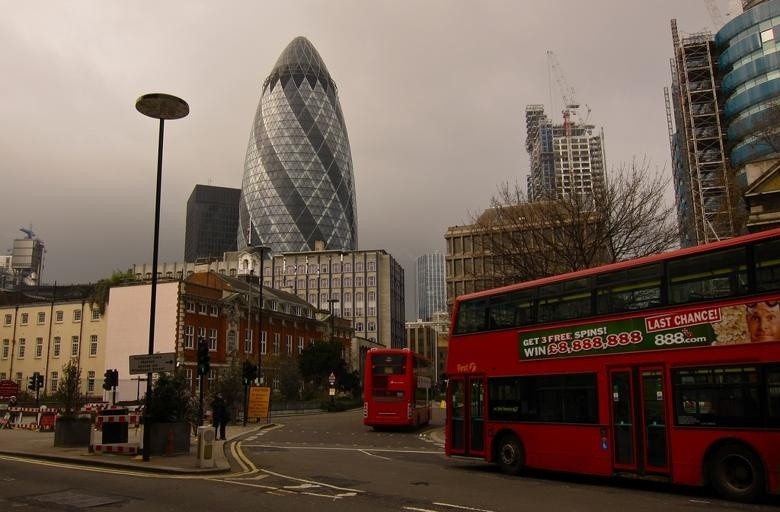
[197,426,216,469]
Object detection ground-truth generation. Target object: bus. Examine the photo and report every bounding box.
[363,348,433,432]
[446,225,780,504]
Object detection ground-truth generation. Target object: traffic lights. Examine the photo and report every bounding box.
[102,368,119,390]
[242,359,258,385]
[28,371,43,389]
[197,339,210,375]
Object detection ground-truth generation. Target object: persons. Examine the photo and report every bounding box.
[745,301,780,343]
[209,390,224,441]
[220,397,232,440]
[128,412,140,437]
